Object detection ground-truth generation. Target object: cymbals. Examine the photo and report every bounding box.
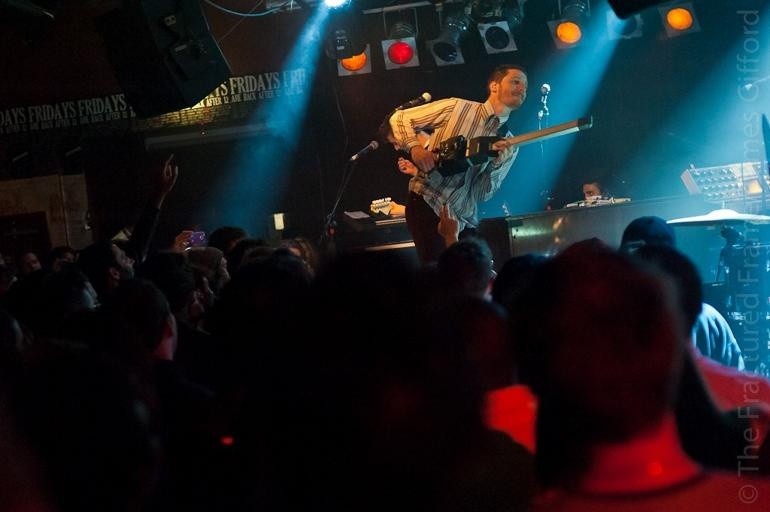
[666,208,770,227]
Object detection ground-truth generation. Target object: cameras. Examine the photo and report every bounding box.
[190,231,205,241]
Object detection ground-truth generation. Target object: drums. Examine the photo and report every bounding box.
[726,244,770,378]
[701,281,726,319]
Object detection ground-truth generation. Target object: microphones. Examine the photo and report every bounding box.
[396,91,432,110]
[349,139,379,163]
[537,84,550,119]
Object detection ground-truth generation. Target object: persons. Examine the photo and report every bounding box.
[0,153,769,511]
[387,63,529,266]
[379,111,434,178]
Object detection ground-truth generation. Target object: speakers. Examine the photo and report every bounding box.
[474,194,709,279]
